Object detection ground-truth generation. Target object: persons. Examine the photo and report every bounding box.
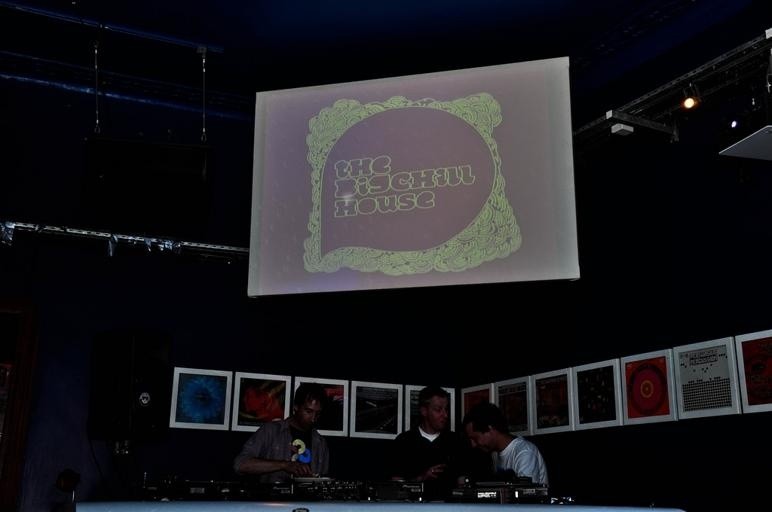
[377,383,492,501]
[457,401,550,490]
[232,381,331,495]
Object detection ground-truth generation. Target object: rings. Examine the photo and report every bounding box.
[431,468,434,473]
